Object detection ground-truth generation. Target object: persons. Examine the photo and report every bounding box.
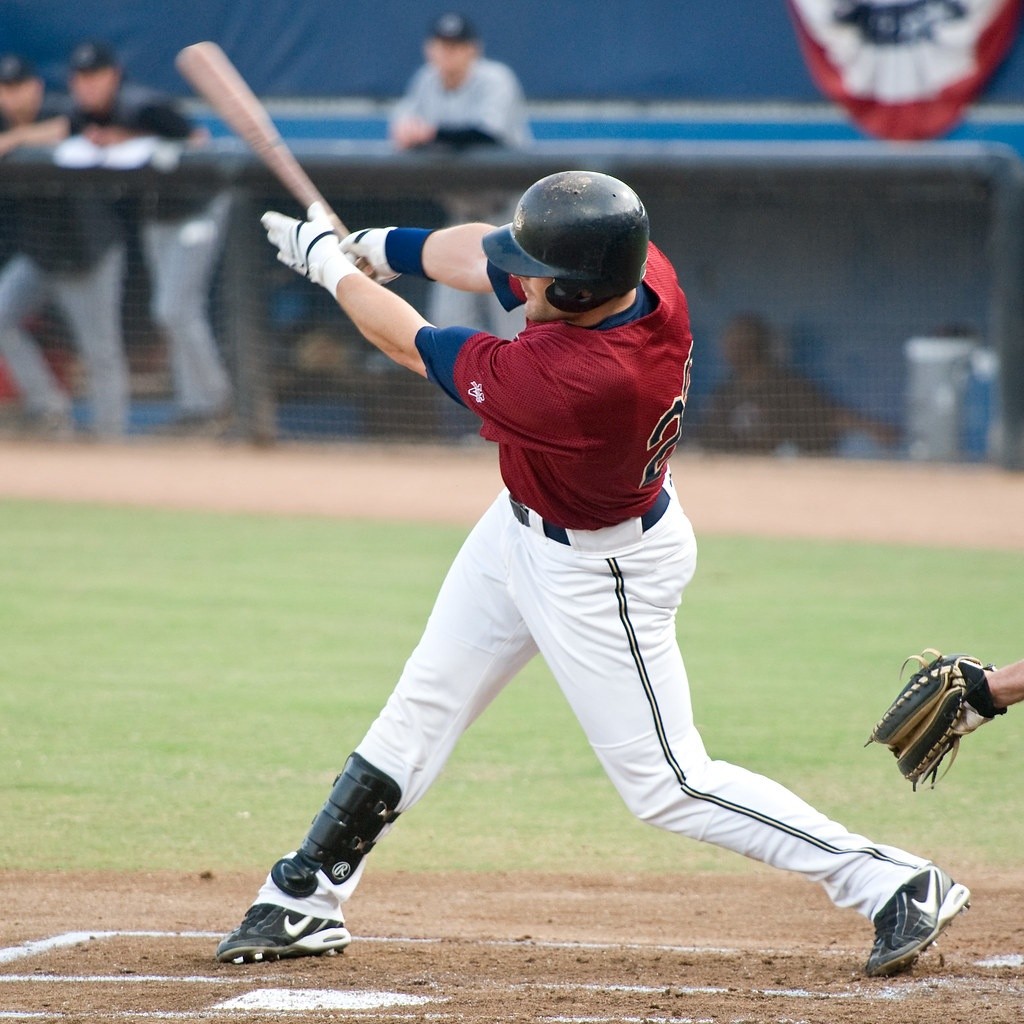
[0,41,232,436]
[391,13,538,444]
[0,53,126,454]
[864,647,1024,792]
[216,170,971,978]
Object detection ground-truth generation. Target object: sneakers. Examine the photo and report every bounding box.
[217,903,352,964]
[864,865,971,977]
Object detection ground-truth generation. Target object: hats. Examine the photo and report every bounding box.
[70,43,113,75]
[0,54,32,84]
[431,11,473,42]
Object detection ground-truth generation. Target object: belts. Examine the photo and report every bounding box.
[509,487,670,546]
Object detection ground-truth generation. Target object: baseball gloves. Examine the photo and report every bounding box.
[862,648,1008,795]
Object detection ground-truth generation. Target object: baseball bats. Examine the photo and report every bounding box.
[177,39,378,280]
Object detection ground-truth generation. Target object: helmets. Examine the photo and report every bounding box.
[480,170,649,313]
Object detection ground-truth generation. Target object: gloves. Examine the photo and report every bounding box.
[339,226,401,285]
[260,202,342,286]
